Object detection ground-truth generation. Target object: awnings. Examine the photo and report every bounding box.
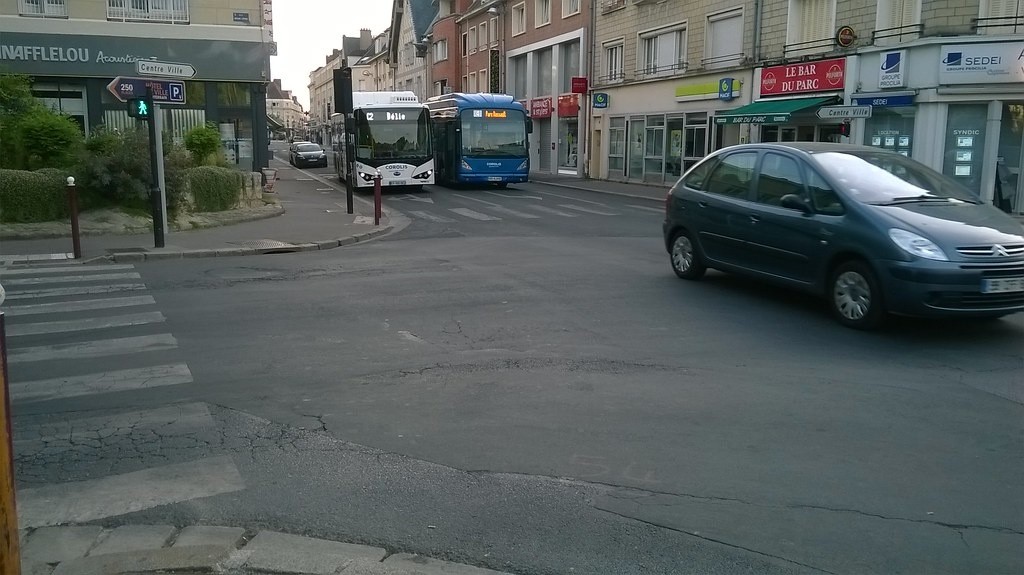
[715,97,837,125]
[267,115,283,131]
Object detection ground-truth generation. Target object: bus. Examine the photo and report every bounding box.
[329,91,437,194]
[423,93,535,192]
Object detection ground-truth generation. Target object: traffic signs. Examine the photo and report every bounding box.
[136,59,198,81]
[105,76,187,106]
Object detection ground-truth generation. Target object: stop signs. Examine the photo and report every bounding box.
[836,26,855,47]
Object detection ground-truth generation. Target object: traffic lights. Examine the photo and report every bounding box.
[127,99,149,119]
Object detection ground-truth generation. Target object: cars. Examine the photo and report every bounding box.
[291,136,303,143]
[289,140,328,169]
[661,139,1023,332]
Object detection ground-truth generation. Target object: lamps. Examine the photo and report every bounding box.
[737,55,749,67]
[362,70,372,76]
[421,37,432,43]
[486,2,504,15]
